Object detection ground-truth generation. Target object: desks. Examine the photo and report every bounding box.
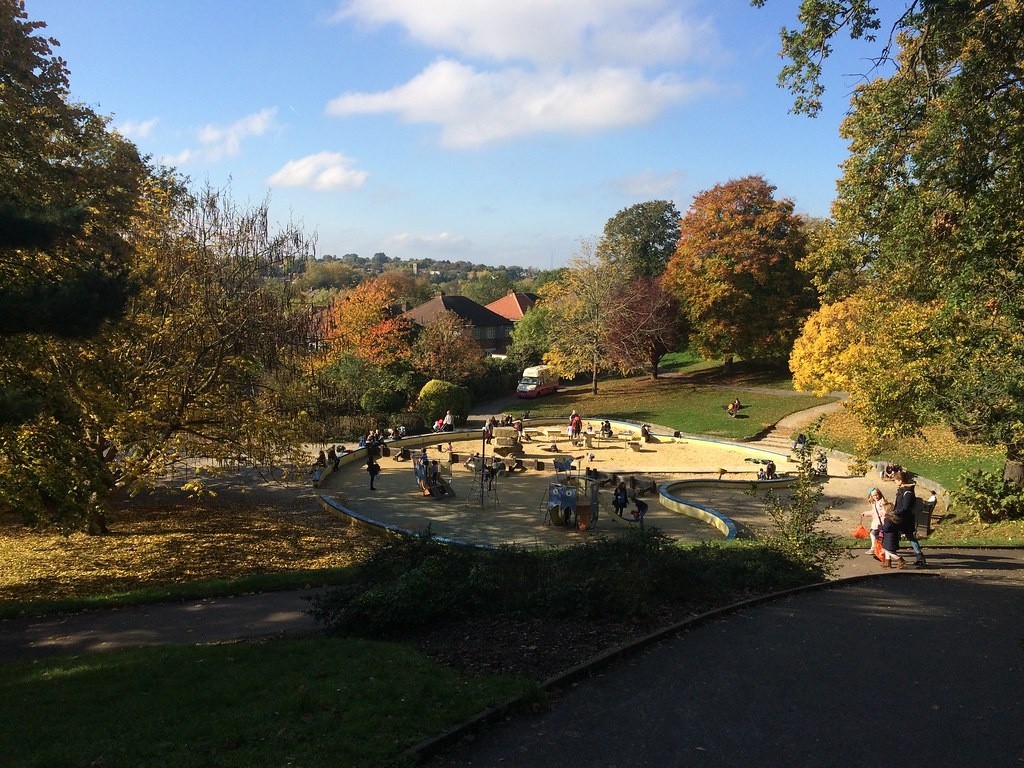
[544,428,561,437]
[618,431,635,440]
[523,428,538,435]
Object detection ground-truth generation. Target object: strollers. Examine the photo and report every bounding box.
[433,419,447,433]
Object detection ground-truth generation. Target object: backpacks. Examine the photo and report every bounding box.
[575,421,580,431]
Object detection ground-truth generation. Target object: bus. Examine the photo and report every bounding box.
[516,365,560,399]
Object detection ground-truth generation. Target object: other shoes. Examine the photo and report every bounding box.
[370,487,376,490]
[336,467,340,471]
[333,467,336,471]
[865,549,875,554]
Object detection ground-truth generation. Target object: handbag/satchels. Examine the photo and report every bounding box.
[514,422,520,429]
[878,524,884,538]
[611,496,616,505]
[851,515,868,539]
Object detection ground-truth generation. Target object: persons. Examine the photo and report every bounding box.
[587,453,595,460]
[605,420,612,437]
[631,497,648,520]
[420,448,428,477]
[734,398,740,417]
[486,414,522,444]
[565,410,583,440]
[860,461,927,569]
[432,461,437,484]
[327,444,340,471]
[313,451,326,466]
[447,441,452,451]
[927,490,936,502]
[367,455,380,491]
[361,429,387,457]
[435,410,455,431]
[794,433,806,450]
[756,461,776,480]
[488,466,495,491]
[641,424,650,443]
[612,482,629,516]
[475,452,480,458]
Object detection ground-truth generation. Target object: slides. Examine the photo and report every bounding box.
[599,493,641,528]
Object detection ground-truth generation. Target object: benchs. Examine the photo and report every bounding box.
[596,431,609,438]
[641,435,646,442]
[923,499,937,516]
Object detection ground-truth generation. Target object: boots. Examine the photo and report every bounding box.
[912,553,926,565]
[895,556,909,569]
[880,559,892,569]
